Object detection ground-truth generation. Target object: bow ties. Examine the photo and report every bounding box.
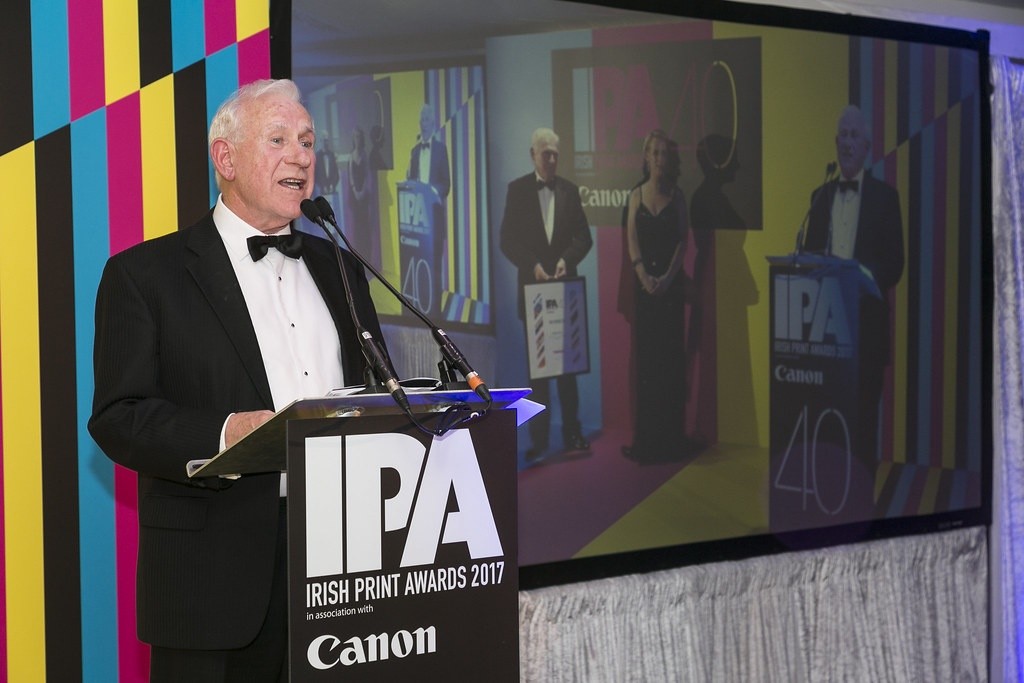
[323,151,328,155]
[837,180,859,192]
[421,142,430,149]
[246,234,302,262]
[537,178,556,190]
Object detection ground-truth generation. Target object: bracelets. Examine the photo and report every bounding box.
[351,185,355,187]
[632,258,642,266]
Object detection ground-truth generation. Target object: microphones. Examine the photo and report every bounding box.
[794,161,838,257]
[299,198,409,412]
[313,195,494,402]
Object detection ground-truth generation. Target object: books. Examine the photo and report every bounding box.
[327,377,442,397]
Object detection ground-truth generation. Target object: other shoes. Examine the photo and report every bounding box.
[524,442,548,456]
[564,432,590,449]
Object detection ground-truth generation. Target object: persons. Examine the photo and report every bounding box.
[87,78,399,683]
[622,128,700,463]
[410,105,451,197]
[315,130,340,193]
[805,105,905,291]
[347,126,371,262]
[501,128,594,460]
